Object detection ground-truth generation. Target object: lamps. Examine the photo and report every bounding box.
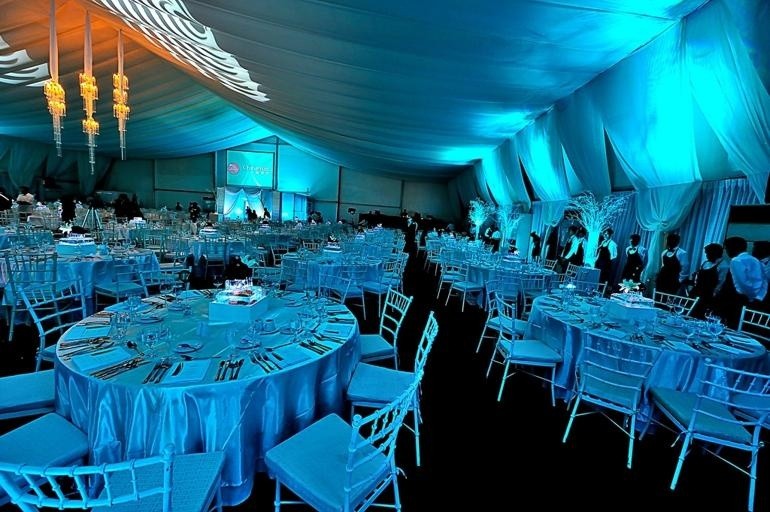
[44,0,130,174]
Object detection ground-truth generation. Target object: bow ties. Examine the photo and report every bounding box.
[629,248,636,250]
[668,248,674,252]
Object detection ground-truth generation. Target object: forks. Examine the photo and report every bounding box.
[143,358,174,386]
[213,348,285,382]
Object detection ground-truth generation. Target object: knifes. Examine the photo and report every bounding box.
[59,310,111,358]
[198,287,213,300]
[89,357,150,380]
[299,309,353,356]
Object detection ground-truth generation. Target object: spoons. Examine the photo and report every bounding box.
[181,354,209,361]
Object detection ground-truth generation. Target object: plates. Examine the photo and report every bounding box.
[174,340,205,352]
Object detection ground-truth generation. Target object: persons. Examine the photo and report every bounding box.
[620,233,649,285]
[689,243,728,320]
[399,212,433,234]
[594,228,618,291]
[752,241,770,314]
[720,237,768,335]
[264,207,271,218]
[176,202,181,210]
[530,230,541,264]
[16,185,34,219]
[575,227,587,267]
[446,220,456,229]
[129,197,143,217]
[491,227,502,252]
[655,234,689,309]
[559,225,578,265]
[483,224,494,242]
[114,193,129,217]
[0,187,10,210]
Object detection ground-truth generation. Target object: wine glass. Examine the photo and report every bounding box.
[0,201,113,262]
[108,279,178,359]
[432,230,549,274]
[213,271,326,356]
[80,208,394,267]
[565,279,726,340]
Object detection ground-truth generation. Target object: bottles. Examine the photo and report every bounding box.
[196,322,208,336]
[183,305,192,316]
